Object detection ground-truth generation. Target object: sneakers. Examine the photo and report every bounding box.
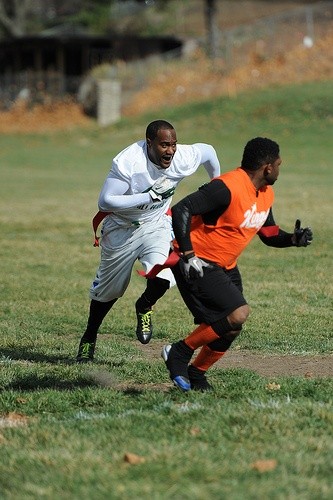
[186,363,214,393]
[134,297,154,345]
[74,335,97,366]
[160,338,195,394]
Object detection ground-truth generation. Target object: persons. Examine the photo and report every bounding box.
[77,120,220,362]
[161,136,313,393]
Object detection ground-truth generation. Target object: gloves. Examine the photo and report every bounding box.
[177,251,223,282]
[293,219,313,247]
[148,173,177,206]
